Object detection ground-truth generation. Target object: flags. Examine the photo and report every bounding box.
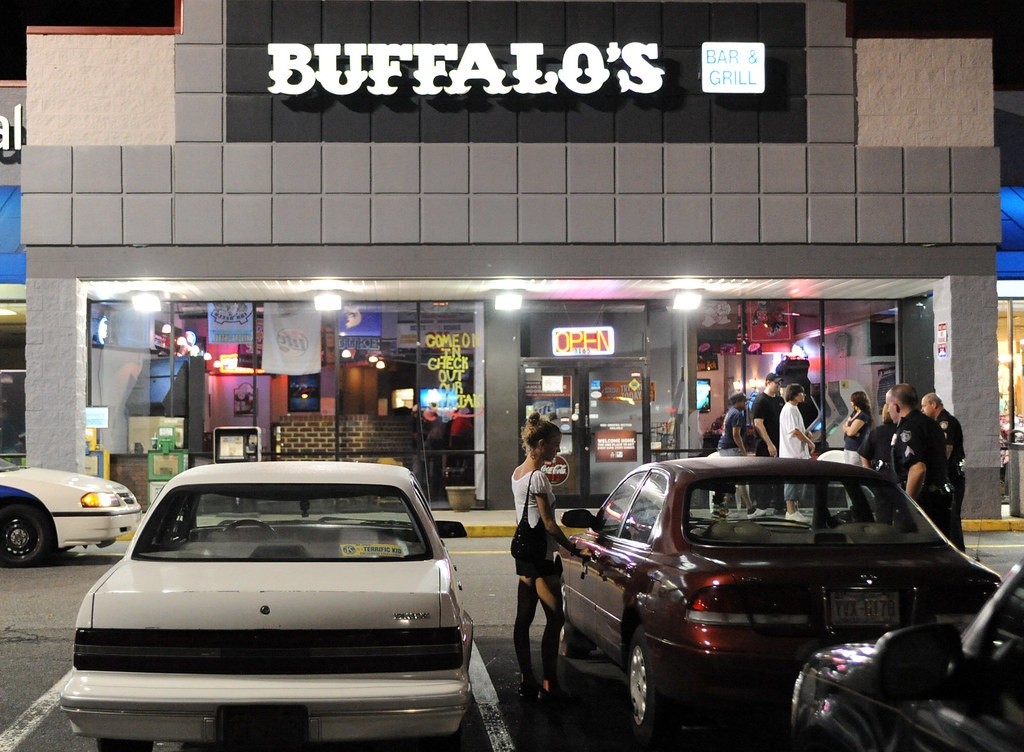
[207,302,794,375]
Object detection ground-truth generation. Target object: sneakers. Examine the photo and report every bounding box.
[785,510,810,523]
[727,512,738,517]
[747,508,766,519]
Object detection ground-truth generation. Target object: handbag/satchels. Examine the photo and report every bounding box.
[509,516,548,562]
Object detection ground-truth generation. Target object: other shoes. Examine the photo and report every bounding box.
[537,688,569,703]
[518,685,539,697]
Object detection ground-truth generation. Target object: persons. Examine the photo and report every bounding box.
[753,373,787,514]
[843,391,874,511]
[780,384,815,523]
[857,402,897,526]
[411,402,474,481]
[511,412,592,702]
[886,384,954,544]
[712,391,767,519]
[920,393,966,554]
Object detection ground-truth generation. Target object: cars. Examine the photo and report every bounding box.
[58,460,474,752]
[785,561,1023,752]
[554,456,1006,752]
[0,455,143,568]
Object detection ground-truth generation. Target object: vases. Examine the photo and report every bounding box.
[445,486,476,513]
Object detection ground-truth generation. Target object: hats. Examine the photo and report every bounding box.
[766,374,784,383]
[730,393,750,401]
